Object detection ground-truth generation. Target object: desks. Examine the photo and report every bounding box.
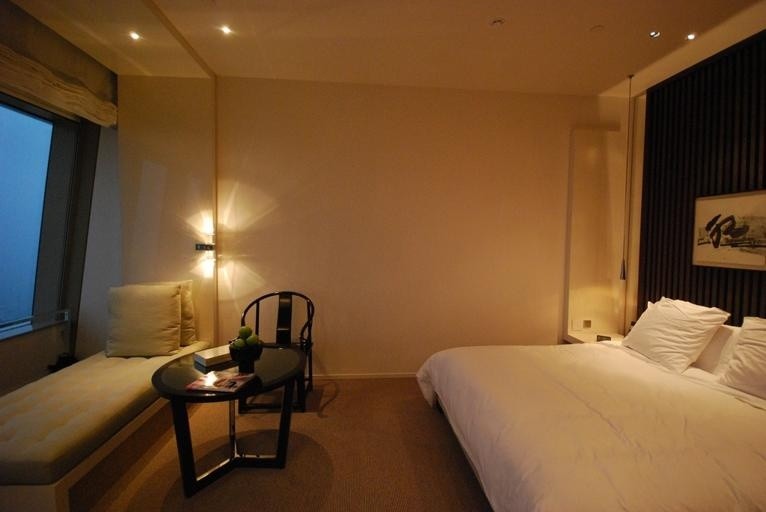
[151,344,308,501]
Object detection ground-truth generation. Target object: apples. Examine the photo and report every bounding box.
[234,326,261,347]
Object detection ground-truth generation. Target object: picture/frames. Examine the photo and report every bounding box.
[691,189,765,272]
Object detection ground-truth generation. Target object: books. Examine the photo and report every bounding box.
[195,344,233,367]
[186,371,256,392]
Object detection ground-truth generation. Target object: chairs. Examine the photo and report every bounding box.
[237,291,315,413]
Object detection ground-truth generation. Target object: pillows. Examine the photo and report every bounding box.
[105,279,197,357]
[619,294,765,401]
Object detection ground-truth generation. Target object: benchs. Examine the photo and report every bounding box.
[0,338,213,509]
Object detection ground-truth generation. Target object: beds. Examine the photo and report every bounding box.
[414,301,765,512]
